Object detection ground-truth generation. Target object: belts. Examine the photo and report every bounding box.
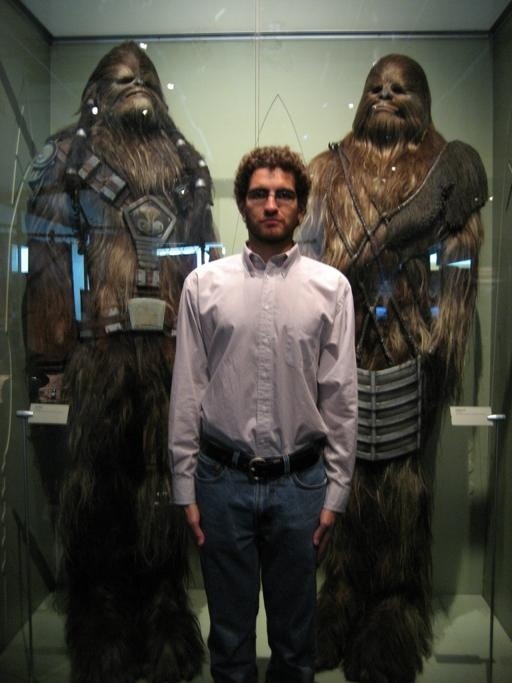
[201,434,321,484]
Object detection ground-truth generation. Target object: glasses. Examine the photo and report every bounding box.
[247,190,296,205]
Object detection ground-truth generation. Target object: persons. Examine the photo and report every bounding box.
[165,143,359,682]
